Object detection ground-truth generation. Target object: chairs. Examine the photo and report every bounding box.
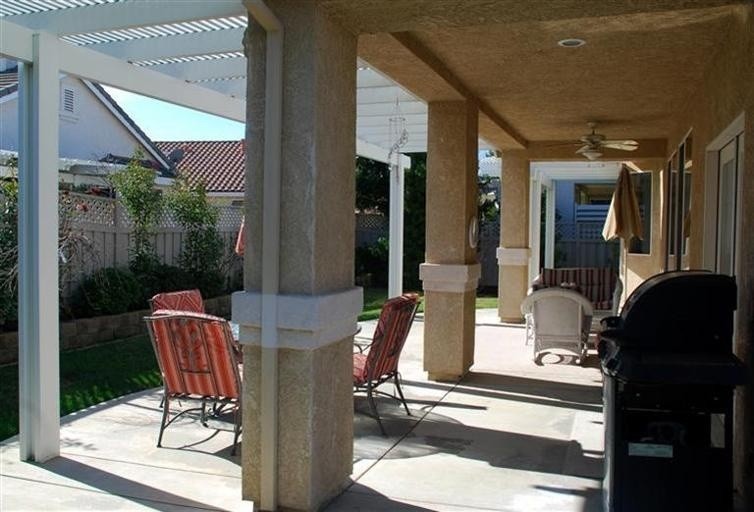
[519,266,623,367]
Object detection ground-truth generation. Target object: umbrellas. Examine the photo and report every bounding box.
[602,163,645,302]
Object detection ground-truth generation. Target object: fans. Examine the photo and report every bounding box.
[545,140,641,150]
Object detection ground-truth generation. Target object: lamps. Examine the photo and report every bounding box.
[575,144,605,161]
